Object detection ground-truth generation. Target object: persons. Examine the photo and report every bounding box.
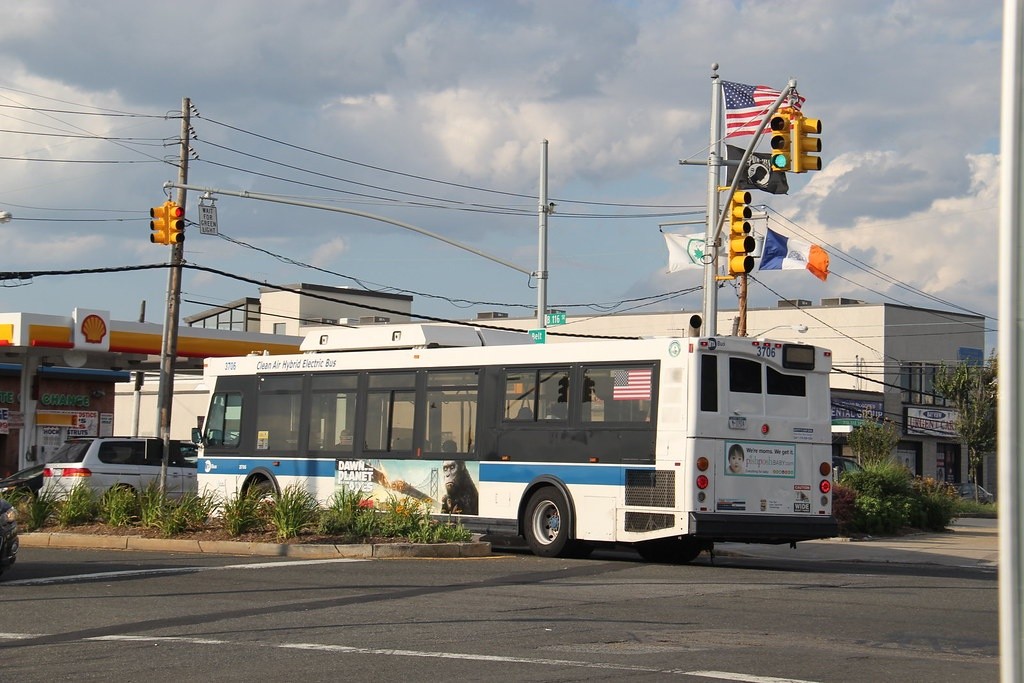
[335,430,352,447]
[726,444,745,473]
[442,440,457,452]
[514,407,533,419]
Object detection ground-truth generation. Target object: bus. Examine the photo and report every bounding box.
[190,322,833,563]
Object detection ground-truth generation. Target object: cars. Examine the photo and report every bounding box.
[0,463,43,505]
[954,483,994,506]
[832,455,868,483]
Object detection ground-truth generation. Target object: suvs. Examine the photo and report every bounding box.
[42,433,200,515]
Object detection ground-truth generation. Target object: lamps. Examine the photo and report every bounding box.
[30,382,41,401]
[92,389,107,399]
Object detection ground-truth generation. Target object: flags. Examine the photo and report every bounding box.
[759,229,828,281]
[723,81,805,138]
[664,231,727,273]
[726,146,789,194]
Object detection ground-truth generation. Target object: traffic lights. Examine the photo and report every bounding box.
[148,202,171,245]
[771,112,794,173]
[726,189,757,276]
[793,115,825,175]
[164,203,186,245]
